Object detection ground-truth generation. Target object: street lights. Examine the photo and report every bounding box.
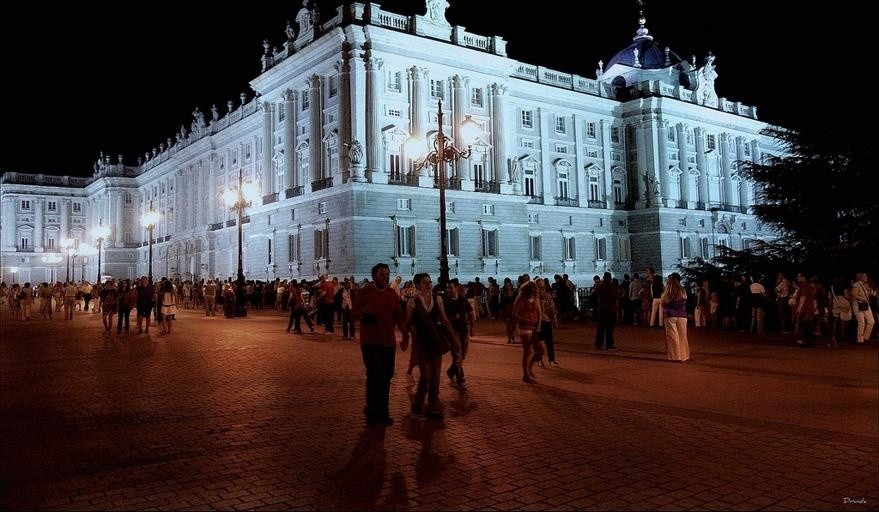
[10,265,17,283]
[140,200,161,284]
[404,97,480,292]
[43,252,63,284]
[61,237,89,284]
[221,166,260,317]
[91,219,111,284]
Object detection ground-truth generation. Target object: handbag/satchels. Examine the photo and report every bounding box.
[788,299,797,306]
[432,326,451,355]
[840,305,849,313]
[858,302,868,311]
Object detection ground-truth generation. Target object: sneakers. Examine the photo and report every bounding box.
[522,371,537,383]
[446,368,467,389]
[549,361,560,366]
[536,361,545,367]
